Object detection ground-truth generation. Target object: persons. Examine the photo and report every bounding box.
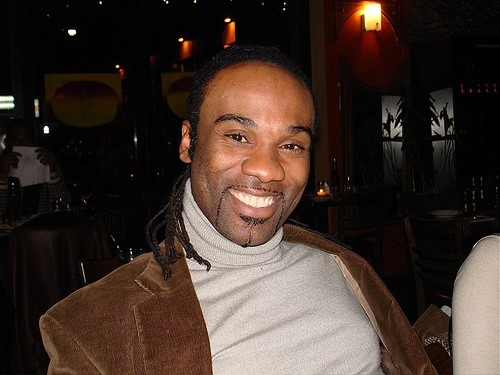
[450,233,500,375]
[40,45,440,375]
[0,118,71,220]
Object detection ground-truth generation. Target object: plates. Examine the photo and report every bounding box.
[429,210,462,217]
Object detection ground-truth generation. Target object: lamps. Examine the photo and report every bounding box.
[353,1,383,32]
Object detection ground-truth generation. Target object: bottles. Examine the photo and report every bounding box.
[344,176,353,191]
[464,176,500,214]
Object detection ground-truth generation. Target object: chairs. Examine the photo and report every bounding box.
[0,210,110,375]
[402,212,468,315]
[328,205,384,275]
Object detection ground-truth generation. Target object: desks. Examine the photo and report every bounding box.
[471,218,500,232]
[296,194,359,231]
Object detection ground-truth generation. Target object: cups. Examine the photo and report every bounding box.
[116,249,146,265]
[316,179,330,196]
